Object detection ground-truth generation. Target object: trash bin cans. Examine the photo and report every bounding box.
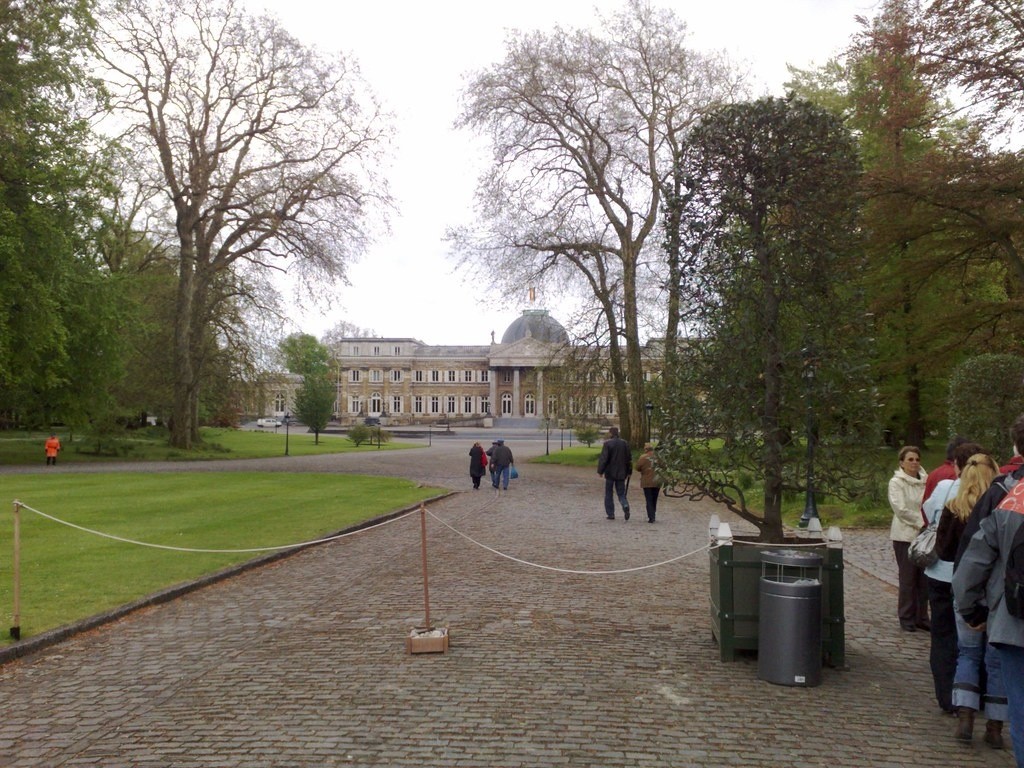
[755,549,826,687]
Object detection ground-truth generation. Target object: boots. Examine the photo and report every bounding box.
[956,706,973,739]
[984,718,1004,748]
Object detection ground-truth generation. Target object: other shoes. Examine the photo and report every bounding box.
[648,518,655,523]
[474,483,478,489]
[915,620,931,631]
[943,704,959,718]
[494,484,498,488]
[504,487,507,490]
[607,515,615,519]
[900,620,917,632]
[623,505,630,520]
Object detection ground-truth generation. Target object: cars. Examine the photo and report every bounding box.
[364,416,382,427]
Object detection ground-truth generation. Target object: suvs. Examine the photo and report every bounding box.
[258,418,282,428]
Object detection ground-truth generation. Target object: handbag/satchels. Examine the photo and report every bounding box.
[511,467,518,479]
[908,524,939,569]
[481,448,488,466]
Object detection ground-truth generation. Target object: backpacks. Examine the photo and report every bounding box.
[1004,521,1024,621]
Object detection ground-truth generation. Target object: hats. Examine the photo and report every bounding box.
[498,439,504,442]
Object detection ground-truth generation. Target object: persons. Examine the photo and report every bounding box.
[45,433,60,466]
[635,443,666,523]
[467,441,486,488]
[887,413,1024,768]
[598,427,633,520]
[486,439,514,490]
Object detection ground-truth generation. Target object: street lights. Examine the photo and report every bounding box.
[799,342,823,528]
[645,402,655,443]
[546,414,551,456]
[284,413,291,456]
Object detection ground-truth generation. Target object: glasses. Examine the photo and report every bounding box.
[904,457,920,462]
